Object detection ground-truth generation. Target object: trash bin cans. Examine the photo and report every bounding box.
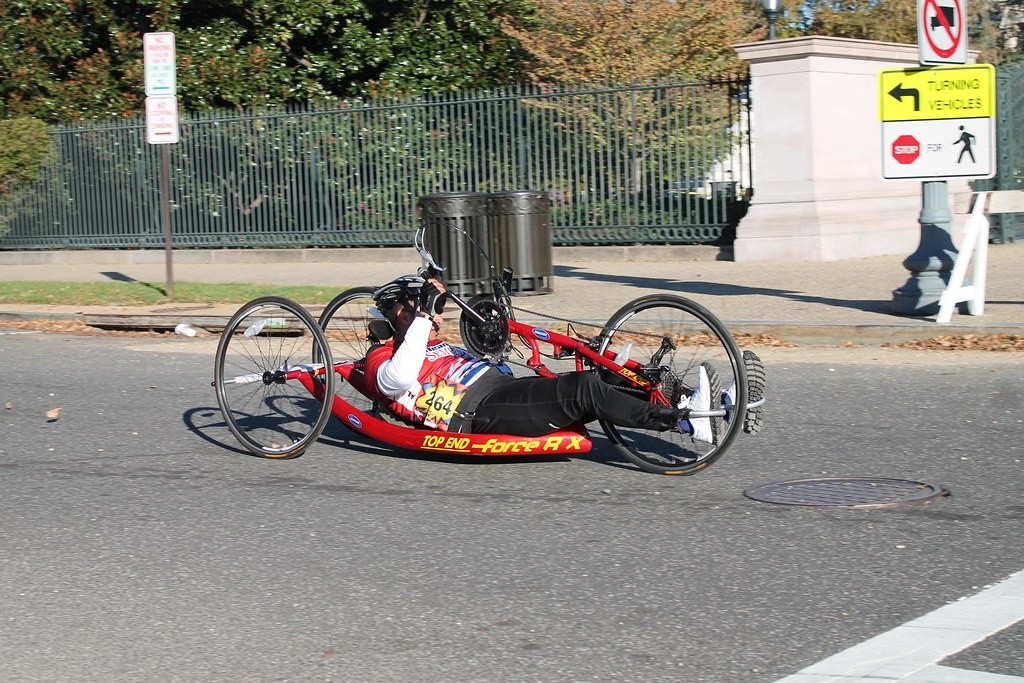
[419,192,496,303]
[489,189,554,297]
[710,181,739,202]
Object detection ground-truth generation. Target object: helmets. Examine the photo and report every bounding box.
[373,274,427,315]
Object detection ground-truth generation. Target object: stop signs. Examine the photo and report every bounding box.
[892,134,920,165]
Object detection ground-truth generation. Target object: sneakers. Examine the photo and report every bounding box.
[723,349,766,435]
[677,362,725,447]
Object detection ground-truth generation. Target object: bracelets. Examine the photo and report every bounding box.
[415,313,433,321]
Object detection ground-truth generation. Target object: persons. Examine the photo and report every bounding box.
[362,273,767,447]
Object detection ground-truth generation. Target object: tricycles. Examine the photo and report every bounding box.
[211,221,766,477]
